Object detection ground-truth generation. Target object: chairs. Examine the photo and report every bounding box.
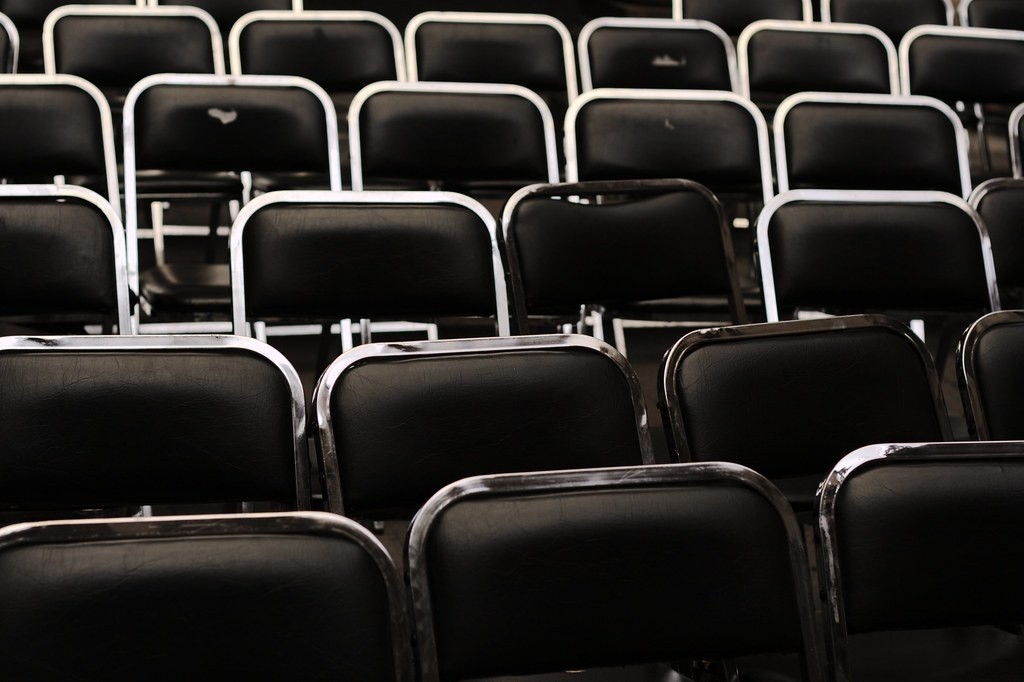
[656,312,953,514]
[1,510,404,682]
[1,331,308,531]
[816,441,1024,680]
[0,0,1024,440]
[403,461,828,682]
[305,335,654,525]
